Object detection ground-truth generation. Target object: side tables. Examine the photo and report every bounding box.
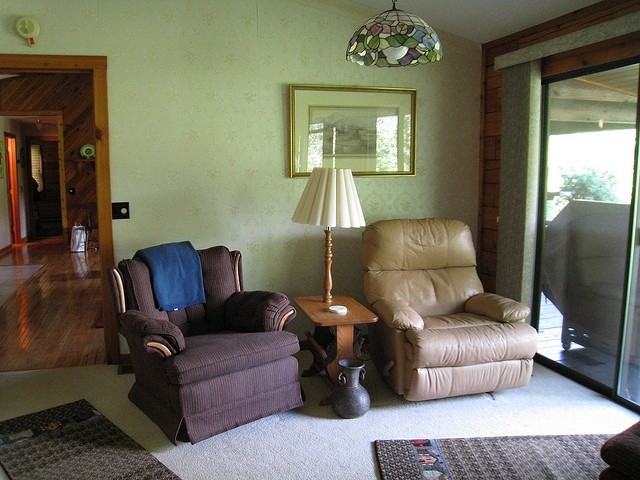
[293,294,381,405]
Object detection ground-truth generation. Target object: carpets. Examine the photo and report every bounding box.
[375,433,623,480]
[1,397,184,480]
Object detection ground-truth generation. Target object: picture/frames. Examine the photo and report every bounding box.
[285,83,417,180]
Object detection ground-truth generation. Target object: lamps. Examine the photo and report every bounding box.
[290,166,367,304]
[345,1,446,71]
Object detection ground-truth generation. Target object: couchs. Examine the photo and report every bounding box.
[361,218,539,401]
[109,244,308,448]
